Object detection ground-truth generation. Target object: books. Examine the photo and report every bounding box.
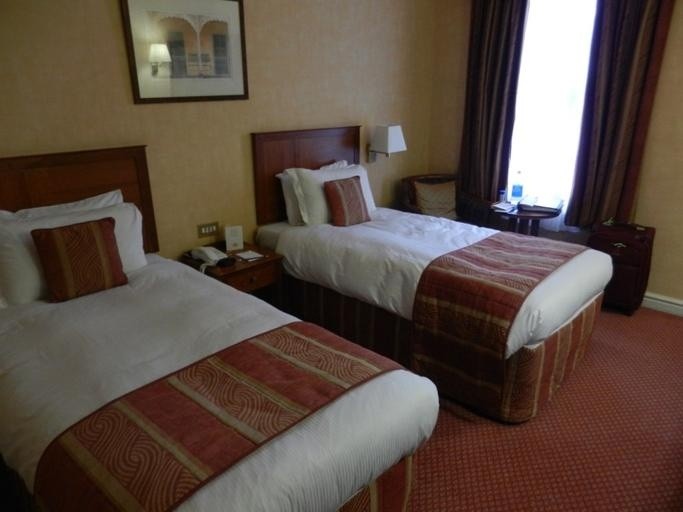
[490,202,513,213]
[518,193,562,212]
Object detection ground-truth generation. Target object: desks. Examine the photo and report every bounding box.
[491,200,560,236]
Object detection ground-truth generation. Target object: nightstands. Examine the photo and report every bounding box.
[183,238,285,294]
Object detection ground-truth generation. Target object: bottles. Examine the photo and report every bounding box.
[499,187,505,202]
[510,171,523,207]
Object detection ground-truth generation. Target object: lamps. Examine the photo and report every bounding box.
[366,123,407,159]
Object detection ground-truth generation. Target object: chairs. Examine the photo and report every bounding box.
[403,172,473,224]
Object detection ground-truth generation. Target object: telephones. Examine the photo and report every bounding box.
[193,246,228,266]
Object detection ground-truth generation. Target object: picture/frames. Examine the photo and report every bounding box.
[119,0,249,104]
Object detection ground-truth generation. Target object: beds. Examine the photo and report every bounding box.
[0,145,442,511]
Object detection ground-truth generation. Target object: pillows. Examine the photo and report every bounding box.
[248,125,613,426]
[2,203,146,304]
[277,160,348,225]
[29,217,129,303]
[286,164,377,227]
[2,188,124,221]
[414,179,459,221]
[323,175,370,227]
